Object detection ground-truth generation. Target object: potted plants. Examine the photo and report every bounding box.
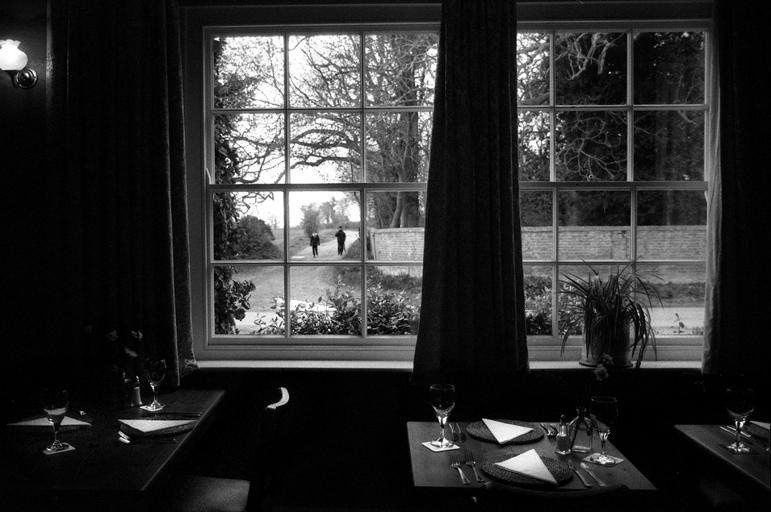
[552,254,666,369]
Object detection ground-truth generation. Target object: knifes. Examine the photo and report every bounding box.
[450,419,468,443]
[568,458,591,487]
[142,411,204,417]
[717,420,771,452]
[579,459,606,485]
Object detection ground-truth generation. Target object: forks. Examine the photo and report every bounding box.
[449,455,472,486]
[464,451,485,482]
[117,429,170,444]
[542,420,570,439]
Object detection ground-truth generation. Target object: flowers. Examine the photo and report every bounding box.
[593,363,609,401]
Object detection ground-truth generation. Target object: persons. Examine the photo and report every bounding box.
[308,229,320,258]
[334,225,345,257]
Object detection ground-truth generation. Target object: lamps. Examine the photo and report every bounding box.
[1,38,38,91]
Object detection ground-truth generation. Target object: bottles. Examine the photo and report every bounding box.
[557,414,572,454]
[129,384,142,408]
[567,407,593,453]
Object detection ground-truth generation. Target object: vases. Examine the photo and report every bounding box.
[589,395,619,421]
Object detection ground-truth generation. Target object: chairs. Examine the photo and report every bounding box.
[476,481,633,511]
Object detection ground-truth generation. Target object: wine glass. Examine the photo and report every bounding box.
[428,382,458,448]
[142,357,170,409]
[589,394,620,465]
[724,389,759,454]
[42,388,72,452]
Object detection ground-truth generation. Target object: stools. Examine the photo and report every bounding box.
[171,476,251,512]
